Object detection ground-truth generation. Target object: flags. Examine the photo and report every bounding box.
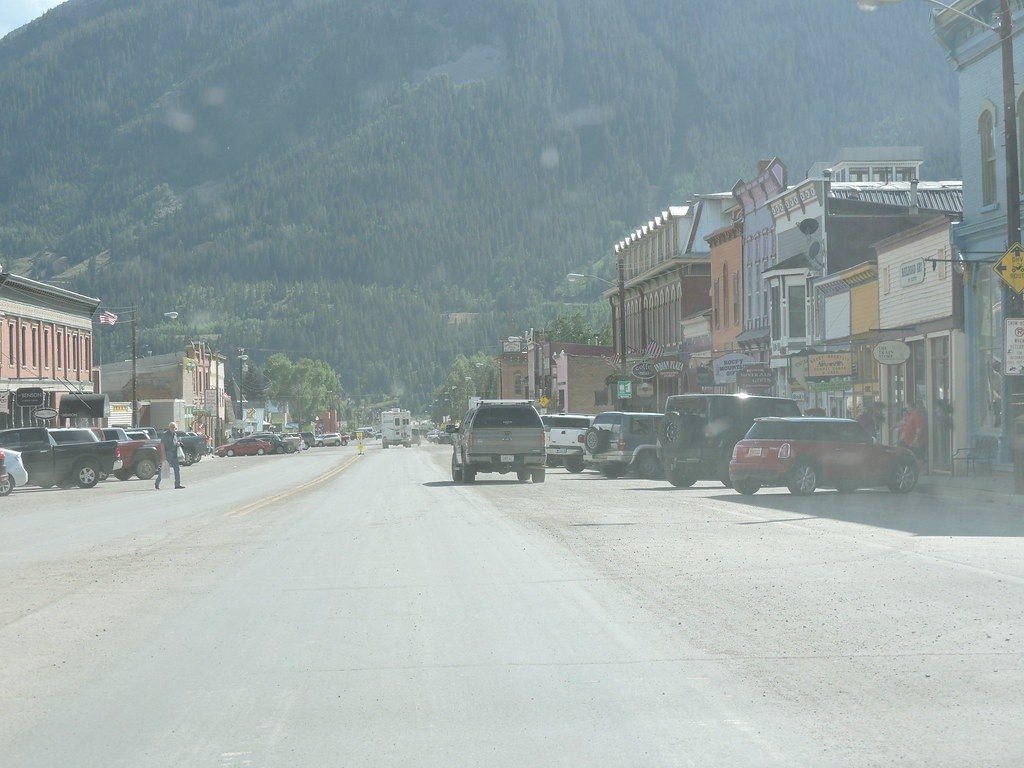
[99,307,118,326]
[605,337,664,365]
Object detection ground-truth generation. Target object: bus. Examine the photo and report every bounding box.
[445,400,547,485]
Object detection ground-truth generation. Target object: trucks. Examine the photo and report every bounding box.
[380,407,412,448]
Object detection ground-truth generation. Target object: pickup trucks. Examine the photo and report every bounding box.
[0,419,208,496]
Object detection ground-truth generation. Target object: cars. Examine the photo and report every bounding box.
[410,426,454,445]
[214,424,382,459]
[728,417,918,496]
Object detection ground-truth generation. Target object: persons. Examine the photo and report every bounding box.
[153,421,185,491]
[854,396,930,477]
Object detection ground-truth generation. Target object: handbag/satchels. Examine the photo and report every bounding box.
[177,444,185,463]
[162,459,170,479]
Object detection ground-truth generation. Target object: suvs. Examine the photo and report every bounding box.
[582,411,664,479]
[660,391,804,488]
[539,413,595,474]
